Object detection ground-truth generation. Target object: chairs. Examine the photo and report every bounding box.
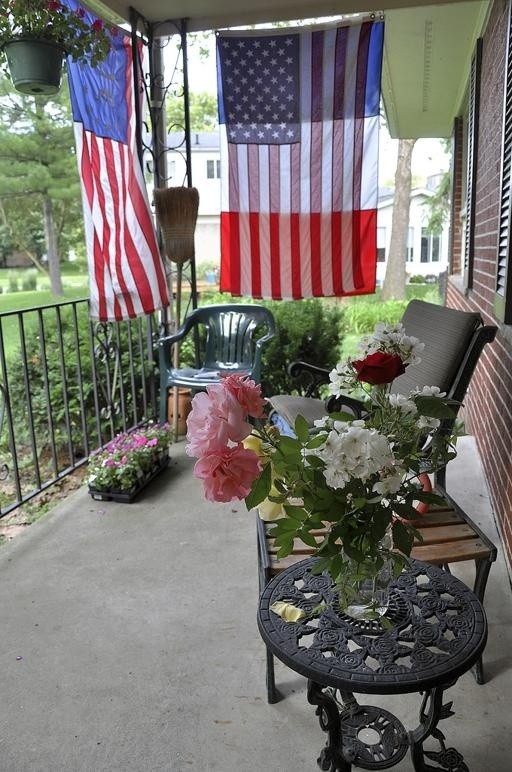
[157,305,276,443]
[263,298,498,517]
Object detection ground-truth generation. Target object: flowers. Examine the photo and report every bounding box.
[184,317,469,633]
[0,0,119,80]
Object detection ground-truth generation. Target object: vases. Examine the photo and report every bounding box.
[6,34,65,95]
[167,387,192,435]
[337,518,396,621]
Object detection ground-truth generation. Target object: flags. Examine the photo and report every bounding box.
[60,0,174,321]
[215,18,385,301]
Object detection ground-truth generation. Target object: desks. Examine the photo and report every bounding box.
[258,556,487,771]
[252,484,499,771]
[258,486,501,705]
